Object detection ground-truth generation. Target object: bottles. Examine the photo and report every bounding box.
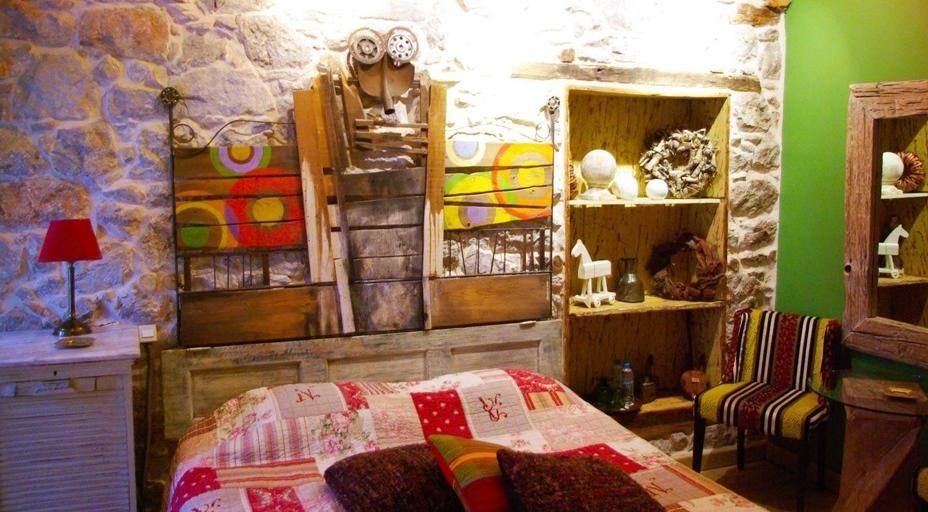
[621,361,635,409]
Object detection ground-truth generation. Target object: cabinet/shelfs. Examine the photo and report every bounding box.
[568,86,731,440]
[877,190,928,287]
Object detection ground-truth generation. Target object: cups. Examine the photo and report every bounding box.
[594,375,623,408]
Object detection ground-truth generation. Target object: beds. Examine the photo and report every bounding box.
[160,318,776,511]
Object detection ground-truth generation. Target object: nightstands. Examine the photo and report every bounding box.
[0,326,140,512]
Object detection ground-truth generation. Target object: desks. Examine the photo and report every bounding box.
[806,366,927,512]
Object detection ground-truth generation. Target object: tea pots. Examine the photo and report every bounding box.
[616,256,648,304]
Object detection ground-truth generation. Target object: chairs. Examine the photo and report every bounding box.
[693,309,842,512]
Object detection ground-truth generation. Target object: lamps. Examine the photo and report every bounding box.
[37,218,102,338]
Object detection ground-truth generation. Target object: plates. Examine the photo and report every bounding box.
[54,338,98,349]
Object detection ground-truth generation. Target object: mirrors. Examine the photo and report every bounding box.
[841,79,928,369]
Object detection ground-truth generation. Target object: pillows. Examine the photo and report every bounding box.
[323,443,465,511]
[495,447,666,511]
[427,434,513,512]
